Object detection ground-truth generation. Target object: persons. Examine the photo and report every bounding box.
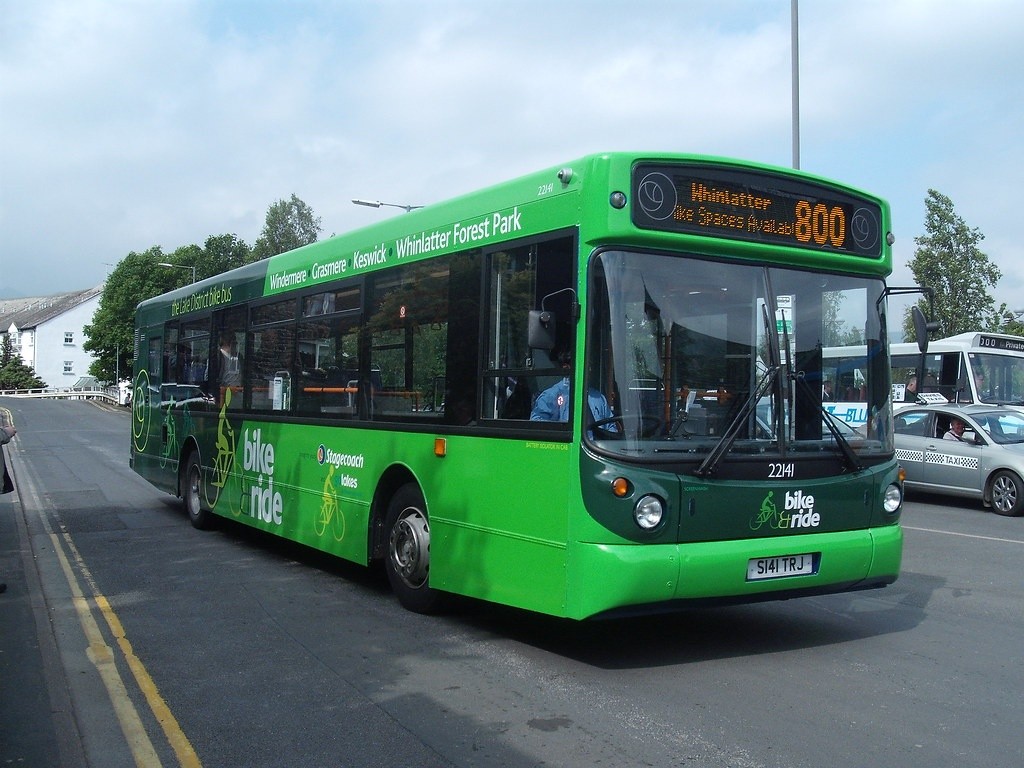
[964,371,989,403]
[528,346,619,433]
[821,380,834,400]
[943,418,973,443]
[205,332,246,409]
[905,375,921,403]
[0,425,17,593]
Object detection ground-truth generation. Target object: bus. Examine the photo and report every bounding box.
[129,151,904,620]
[766,332,1024,434]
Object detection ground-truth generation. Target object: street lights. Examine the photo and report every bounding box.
[158,263,195,372]
[113,341,119,390]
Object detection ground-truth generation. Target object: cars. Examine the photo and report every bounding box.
[855,405,1024,516]
[158,382,215,407]
[674,397,866,442]
[299,338,382,406]
[180,363,206,384]
